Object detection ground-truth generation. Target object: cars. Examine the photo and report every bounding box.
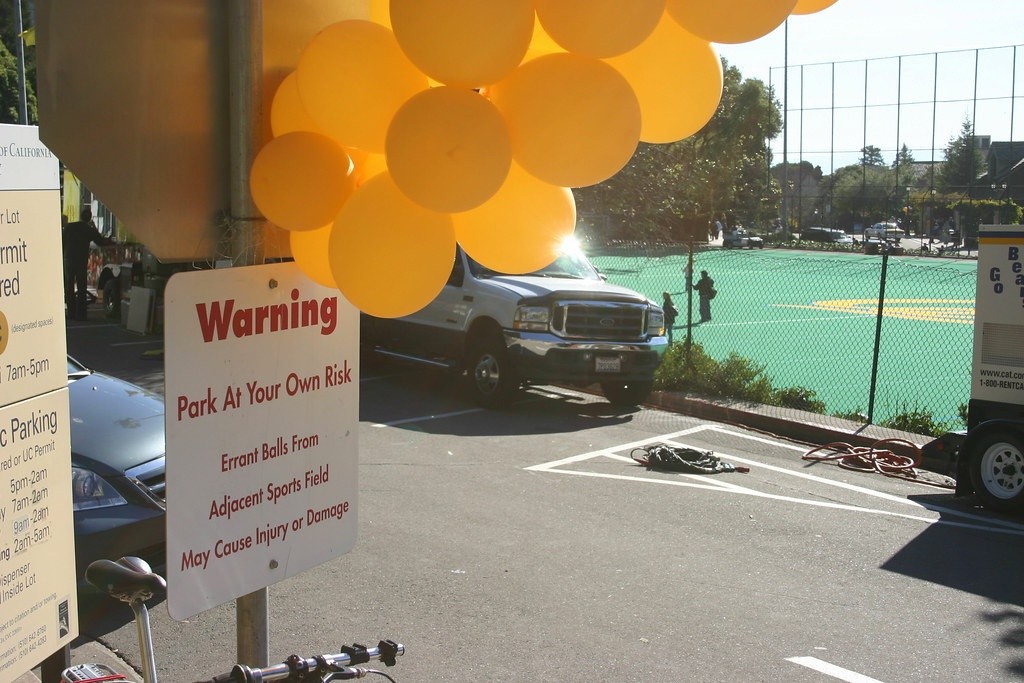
[64,353,168,596]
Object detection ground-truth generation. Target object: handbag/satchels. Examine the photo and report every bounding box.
[670,306,678,317]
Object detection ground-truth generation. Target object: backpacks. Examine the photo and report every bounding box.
[708,285,717,299]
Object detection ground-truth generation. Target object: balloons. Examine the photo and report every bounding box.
[250,1,838,319]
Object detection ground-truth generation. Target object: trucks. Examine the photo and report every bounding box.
[722,231,764,249]
[955,226,1024,512]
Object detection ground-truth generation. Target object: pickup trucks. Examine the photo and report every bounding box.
[865,223,905,243]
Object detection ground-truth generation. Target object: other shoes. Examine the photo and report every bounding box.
[701,318,709,321]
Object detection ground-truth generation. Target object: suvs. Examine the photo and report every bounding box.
[366,229,672,414]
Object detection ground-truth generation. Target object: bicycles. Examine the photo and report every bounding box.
[60,555,408,683]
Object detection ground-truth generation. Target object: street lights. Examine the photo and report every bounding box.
[991,183,1007,225]
[931,188,936,247]
[905,185,912,239]
[782,179,796,241]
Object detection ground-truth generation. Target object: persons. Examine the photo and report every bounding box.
[708,221,722,241]
[852,238,859,246]
[921,243,928,251]
[681,263,694,291]
[692,270,714,322]
[662,291,674,345]
[61,209,113,321]
